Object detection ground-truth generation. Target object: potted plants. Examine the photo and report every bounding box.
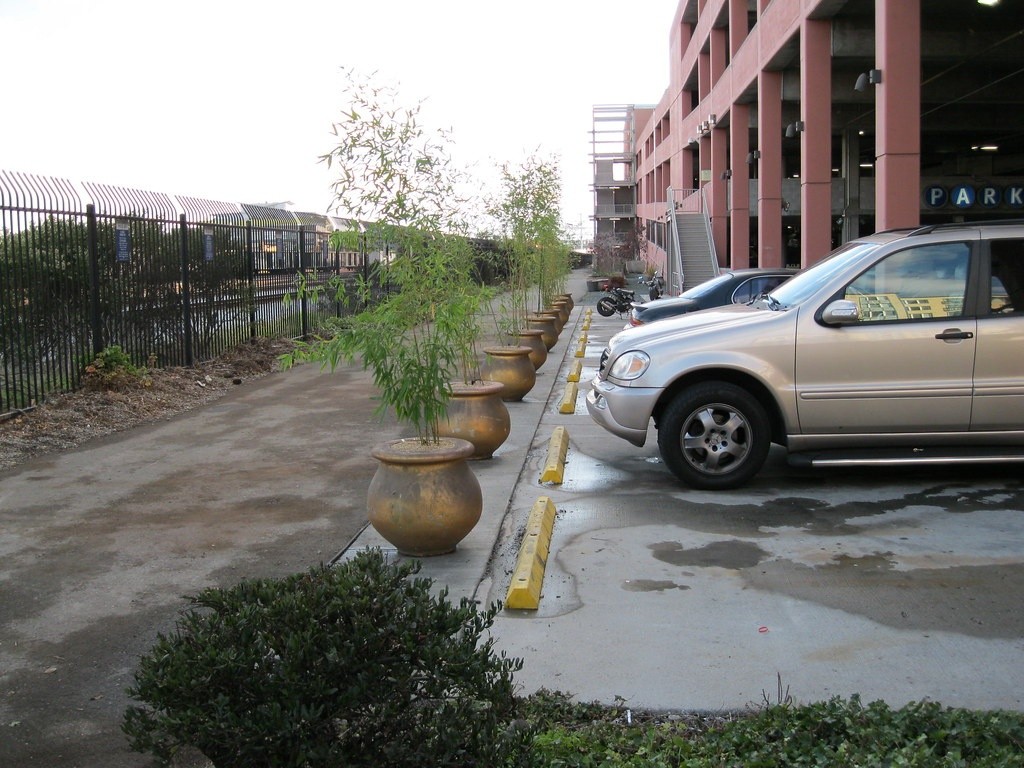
[550,217,574,311]
[521,181,563,337]
[528,187,569,326]
[541,207,571,316]
[275,49,482,559]
[490,143,559,353]
[484,200,548,372]
[438,171,536,403]
[371,123,511,461]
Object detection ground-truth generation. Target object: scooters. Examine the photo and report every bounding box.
[596,270,664,317]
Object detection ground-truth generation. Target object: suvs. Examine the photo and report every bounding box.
[584,217,1023,491]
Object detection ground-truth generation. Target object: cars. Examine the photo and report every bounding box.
[623,267,803,330]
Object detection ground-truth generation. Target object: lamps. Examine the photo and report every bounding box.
[854,70,881,94]
[719,170,732,180]
[745,151,760,163]
[786,121,804,137]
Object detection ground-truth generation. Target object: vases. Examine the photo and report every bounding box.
[587,278,611,292]
